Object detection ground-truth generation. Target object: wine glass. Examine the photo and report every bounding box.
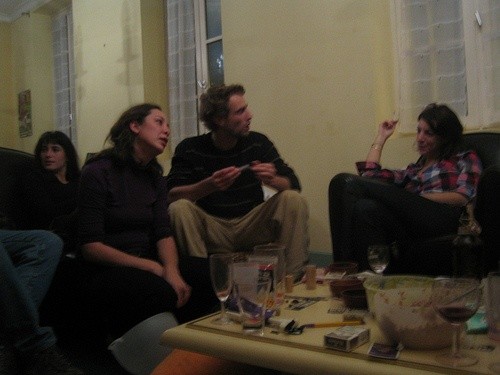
[432,277,481,367]
[487,274,500,375]
[208,253,234,325]
[365,244,392,277]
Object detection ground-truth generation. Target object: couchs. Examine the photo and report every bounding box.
[0,147,78,326]
[328,132,500,279]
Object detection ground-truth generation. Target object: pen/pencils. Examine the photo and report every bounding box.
[239,163,251,172]
[302,320,364,329]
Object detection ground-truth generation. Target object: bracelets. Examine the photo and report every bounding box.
[371,143,383,149]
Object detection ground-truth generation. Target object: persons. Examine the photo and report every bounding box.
[0,104,223,375]
[343,103,481,278]
[166,81,310,319]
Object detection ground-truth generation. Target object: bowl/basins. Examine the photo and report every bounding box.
[362,275,441,317]
[330,280,362,296]
[375,288,471,351]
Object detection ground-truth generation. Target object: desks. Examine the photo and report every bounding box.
[159,277,500,375]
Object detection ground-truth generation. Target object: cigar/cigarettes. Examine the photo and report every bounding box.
[391,112,396,121]
[239,165,249,170]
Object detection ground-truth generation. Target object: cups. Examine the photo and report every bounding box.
[344,291,368,309]
[253,245,286,313]
[235,276,271,338]
[231,262,259,296]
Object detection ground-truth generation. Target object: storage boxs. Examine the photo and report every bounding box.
[324,326,371,352]
